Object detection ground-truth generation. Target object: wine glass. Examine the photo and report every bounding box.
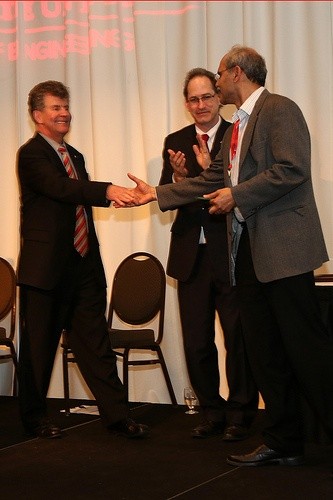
[184,387,199,414]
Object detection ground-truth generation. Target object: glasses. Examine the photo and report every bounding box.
[214,65,244,81]
[187,92,216,105]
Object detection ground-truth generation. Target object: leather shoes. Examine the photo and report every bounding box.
[226,443,305,466]
[123,417,151,438]
[36,418,63,438]
[192,417,224,438]
[223,424,248,440]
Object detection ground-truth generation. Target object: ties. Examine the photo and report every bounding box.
[58,145,89,258]
[228,120,240,169]
[199,134,209,176]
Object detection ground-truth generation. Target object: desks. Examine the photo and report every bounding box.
[304,275,333,449]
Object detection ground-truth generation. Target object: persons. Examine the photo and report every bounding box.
[113,47,330,467]
[19,80,150,439]
[159,68,258,442]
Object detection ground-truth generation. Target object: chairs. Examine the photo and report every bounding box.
[60,250,179,417]
[0,256,20,405]
